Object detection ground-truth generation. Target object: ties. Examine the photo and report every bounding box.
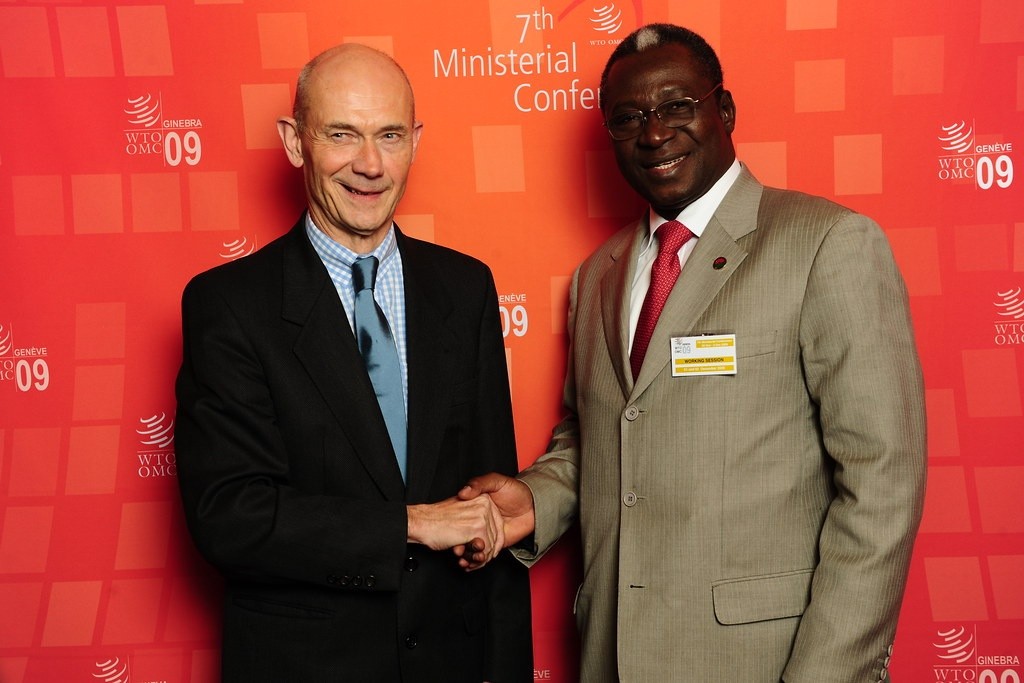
[351,255,407,486]
[629,220,695,385]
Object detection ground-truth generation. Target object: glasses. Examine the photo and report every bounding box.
[603,82,722,140]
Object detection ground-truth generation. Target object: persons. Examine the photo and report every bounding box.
[452,23,927,683]
[174,43,534,683]
